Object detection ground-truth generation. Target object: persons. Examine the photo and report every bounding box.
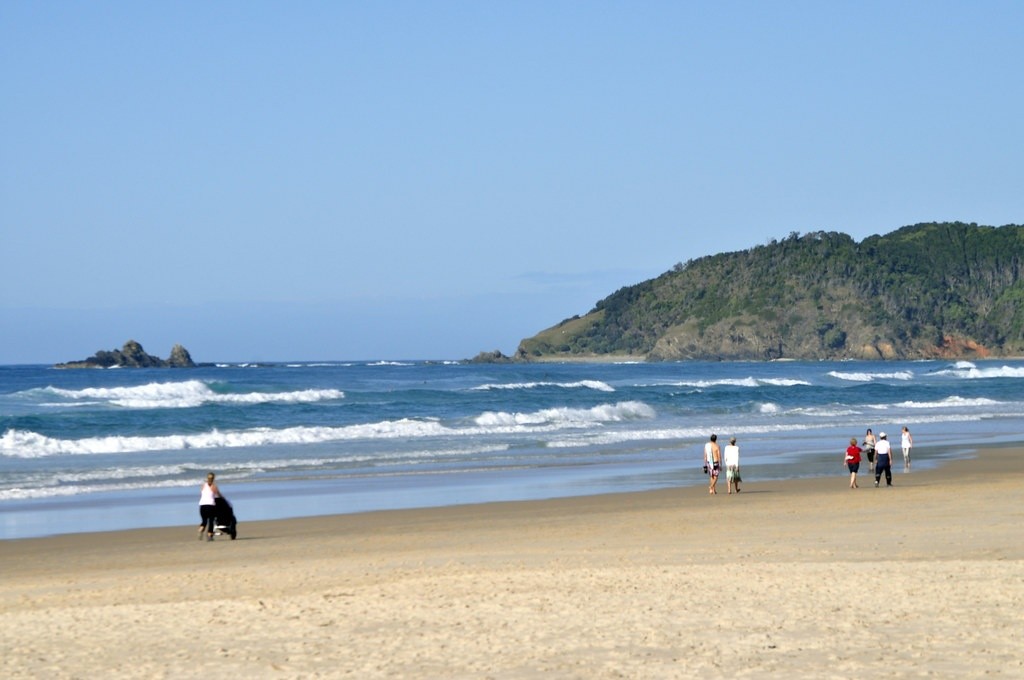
[724,437,743,495]
[844,429,893,489]
[901,427,913,473]
[196,473,221,541]
[703,435,722,495]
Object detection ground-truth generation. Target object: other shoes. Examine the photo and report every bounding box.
[887,484,893,487]
[875,481,879,488]
[198,526,204,539]
[207,533,214,541]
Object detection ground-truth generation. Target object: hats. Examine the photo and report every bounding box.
[879,432,887,438]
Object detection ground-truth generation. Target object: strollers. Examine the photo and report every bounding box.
[210,497,237,541]
